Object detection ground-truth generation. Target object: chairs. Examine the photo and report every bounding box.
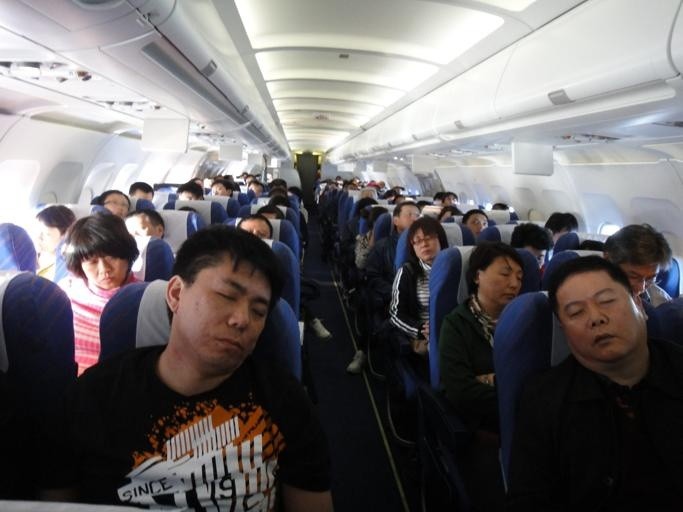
[316,178,682,512]
[0,179,305,511]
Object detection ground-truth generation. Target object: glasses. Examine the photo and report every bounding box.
[410,233,441,245]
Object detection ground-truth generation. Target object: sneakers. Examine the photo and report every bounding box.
[310,316,332,342]
[345,350,370,378]
